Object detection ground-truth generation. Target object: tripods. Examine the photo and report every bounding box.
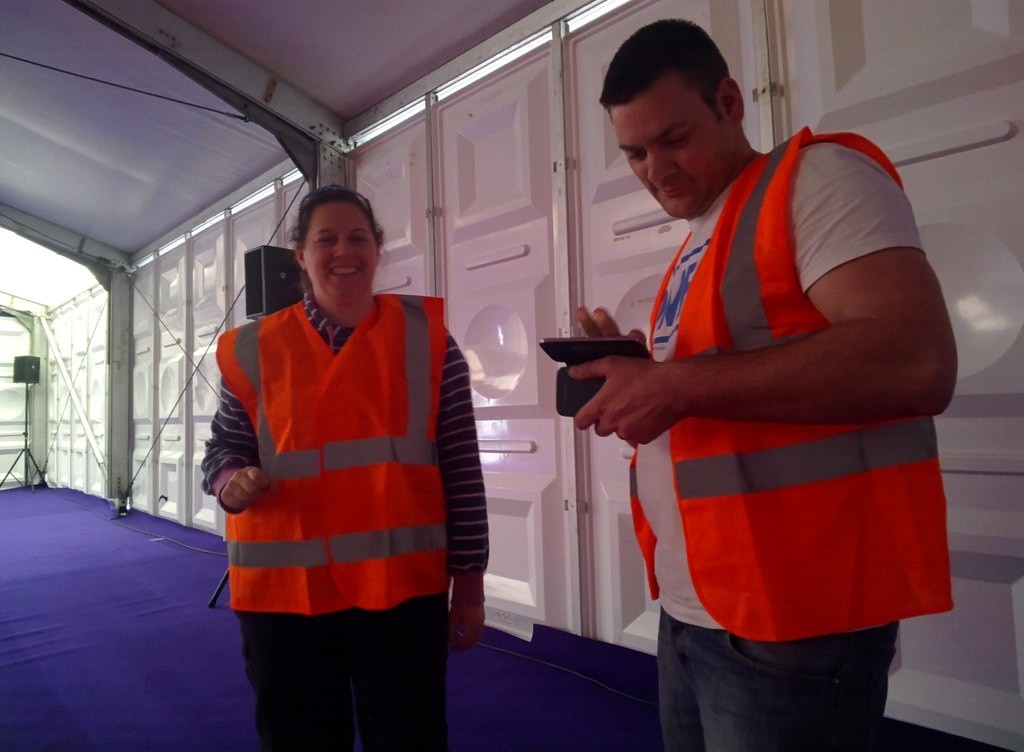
[0,383,48,493]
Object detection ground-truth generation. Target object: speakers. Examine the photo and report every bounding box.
[245,245,304,319]
[13,356,40,383]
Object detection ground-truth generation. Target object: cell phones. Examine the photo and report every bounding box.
[539,337,651,362]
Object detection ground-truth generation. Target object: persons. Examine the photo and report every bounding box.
[201,184,490,752]
[574,18,960,752]
